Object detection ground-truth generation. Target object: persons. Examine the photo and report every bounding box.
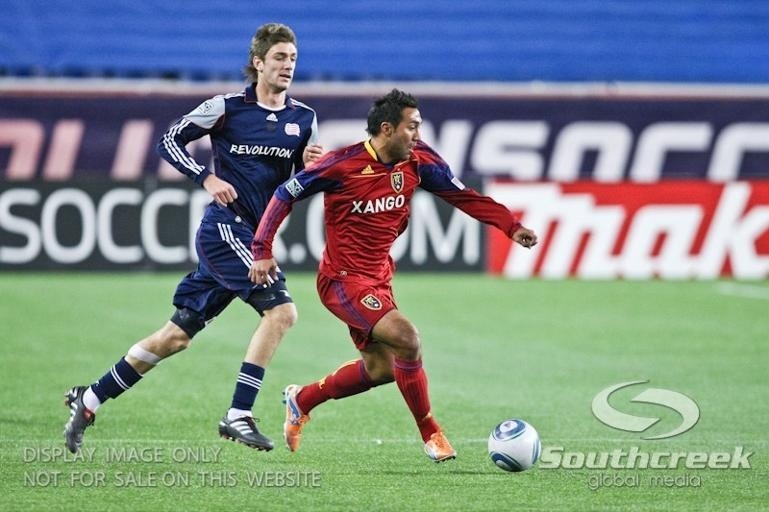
[247,89,538,464]
[64,23,325,455]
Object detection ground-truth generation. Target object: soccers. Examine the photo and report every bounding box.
[489,420,540,471]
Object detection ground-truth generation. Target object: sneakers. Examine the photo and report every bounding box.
[280,383,311,452]
[62,384,97,454]
[423,431,458,463]
[217,411,276,453]
